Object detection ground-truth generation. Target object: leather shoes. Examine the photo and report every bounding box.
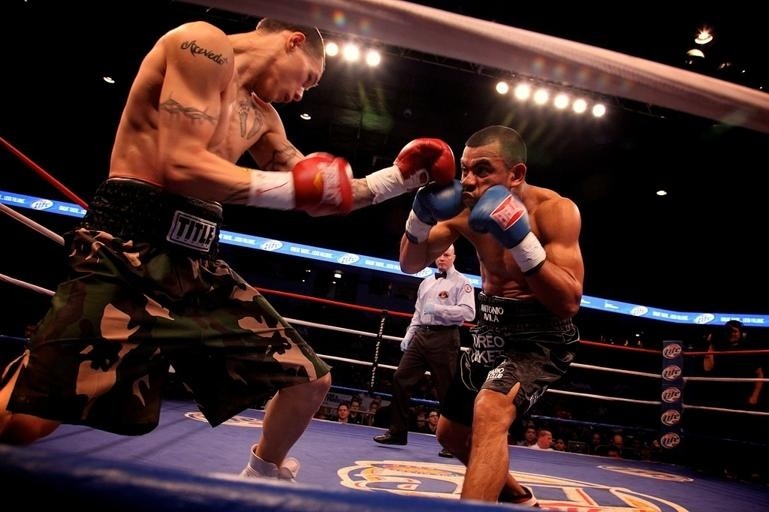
[438,448,457,458]
[373,429,408,446]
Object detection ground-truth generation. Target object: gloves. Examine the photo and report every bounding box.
[400,332,415,351]
[467,182,548,276]
[404,177,465,245]
[364,136,457,206]
[422,302,437,316]
[245,150,357,219]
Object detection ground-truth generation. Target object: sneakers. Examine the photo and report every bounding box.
[499,482,542,508]
[237,440,300,486]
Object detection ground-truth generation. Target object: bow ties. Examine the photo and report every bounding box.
[434,271,448,280]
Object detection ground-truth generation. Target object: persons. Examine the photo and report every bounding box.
[398,125,586,510]
[373,242,476,458]
[688,320,769,484]
[320,390,647,459]
[1,14,456,485]
